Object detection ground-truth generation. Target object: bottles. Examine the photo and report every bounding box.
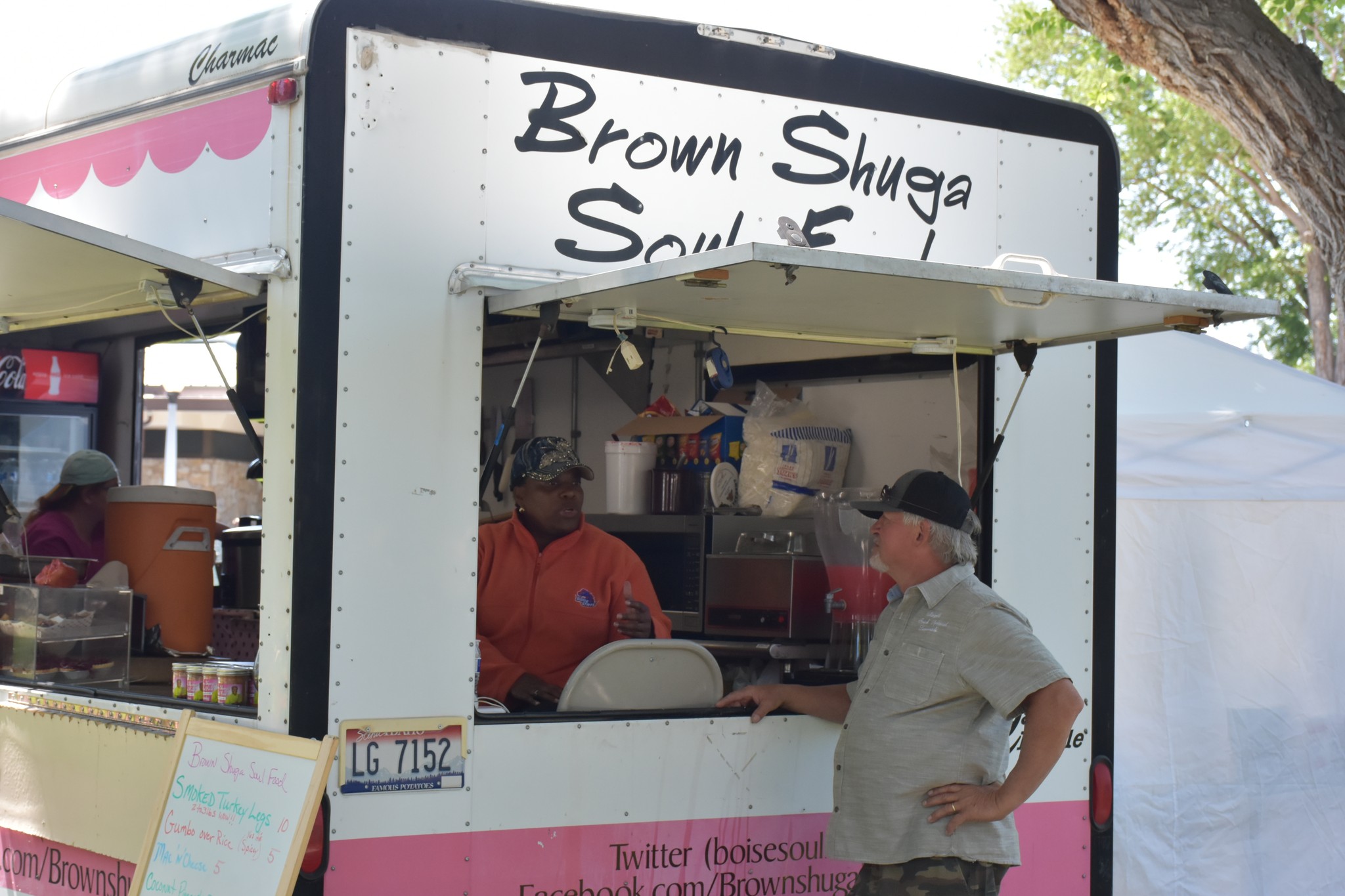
[0,458,63,507]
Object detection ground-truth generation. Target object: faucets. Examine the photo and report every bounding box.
[824,588,846,614]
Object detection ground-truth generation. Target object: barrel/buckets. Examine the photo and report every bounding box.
[105,484,219,656]
[650,467,694,514]
[221,515,262,610]
[604,440,658,516]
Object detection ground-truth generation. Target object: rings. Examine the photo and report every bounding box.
[533,690,539,696]
[951,802,957,813]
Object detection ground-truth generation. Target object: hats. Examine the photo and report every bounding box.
[851,468,975,534]
[509,435,595,490]
[60,448,117,486]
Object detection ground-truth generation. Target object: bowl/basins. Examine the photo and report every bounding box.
[0,609,96,639]
[0,667,115,681]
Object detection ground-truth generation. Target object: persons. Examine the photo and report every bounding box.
[478,434,674,714]
[715,468,1086,896]
[22,449,121,586]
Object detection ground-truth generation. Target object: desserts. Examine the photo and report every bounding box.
[0,657,114,682]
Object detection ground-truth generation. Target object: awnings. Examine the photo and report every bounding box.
[0,191,265,334]
[480,241,1286,368]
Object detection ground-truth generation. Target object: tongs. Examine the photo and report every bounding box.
[705,504,762,516]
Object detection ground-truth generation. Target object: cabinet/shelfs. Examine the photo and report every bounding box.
[0,582,133,690]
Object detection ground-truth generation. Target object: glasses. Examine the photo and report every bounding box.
[880,484,940,514]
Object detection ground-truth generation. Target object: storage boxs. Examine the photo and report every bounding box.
[615,402,747,473]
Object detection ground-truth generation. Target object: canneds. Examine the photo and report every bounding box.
[171,662,258,708]
